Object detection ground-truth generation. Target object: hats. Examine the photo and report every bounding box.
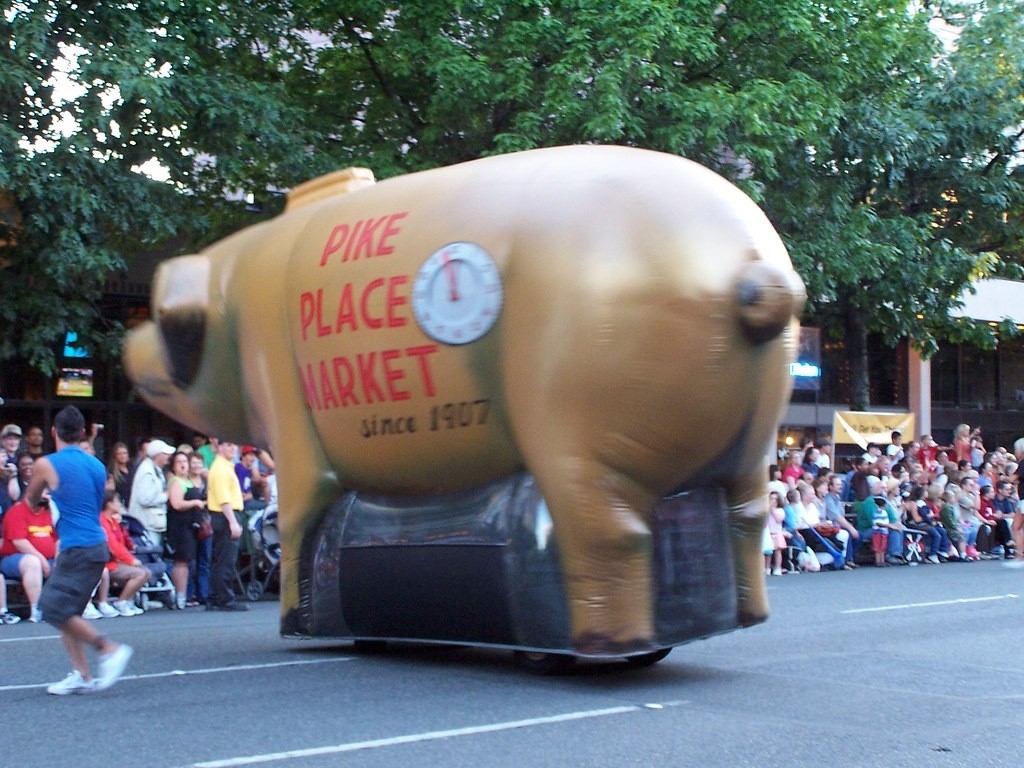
[816,437,832,447]
[147,439,176,457]
[241,444,258,454]
[2,424,22,435]
[893,465,906,472]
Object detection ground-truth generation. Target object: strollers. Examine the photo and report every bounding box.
[233,500,284,602]
[115,511,178,611]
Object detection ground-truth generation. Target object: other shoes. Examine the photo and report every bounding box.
[185,596,201,606]
[171,599,190,610]
[772,569,782,576]
[93,641,131,691]
[841,565,852,570]
[846,560,860,568]
[45,668,93,695]
[149,600,165,609]
[875,545,1016,565]
[206,599,250,611]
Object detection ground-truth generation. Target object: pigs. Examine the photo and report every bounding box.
[123,139,814,656]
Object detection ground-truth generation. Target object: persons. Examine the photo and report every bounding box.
[0,424,60,624]
[763,423,1024,575]
[82,434,281,617]
[25,406,136,698]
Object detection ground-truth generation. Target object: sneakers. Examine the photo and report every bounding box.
[112,600,143,616]
[83,604,103,620]
[0,611,20,625]
[96,602,119,617]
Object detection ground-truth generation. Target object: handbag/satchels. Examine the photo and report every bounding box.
[798,545,821,572]
[813,524,839,538]
[196,508,215,539]
